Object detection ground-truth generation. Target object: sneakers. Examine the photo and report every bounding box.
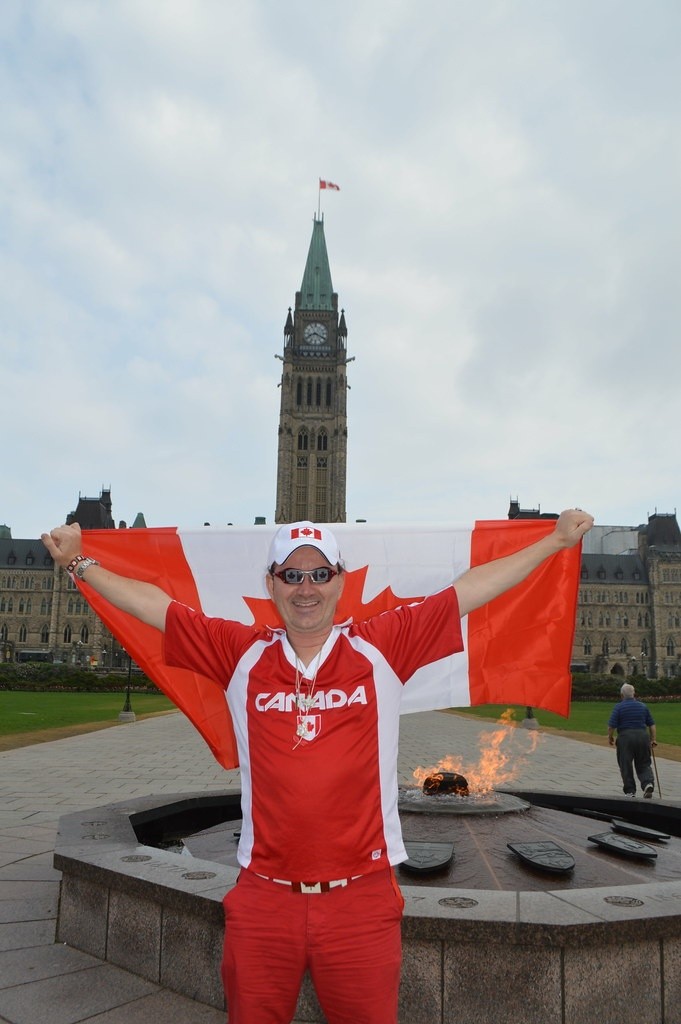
[643,783,654,798]
[625,793,635,797]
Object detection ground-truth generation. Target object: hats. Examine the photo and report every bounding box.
[266,520,341,570]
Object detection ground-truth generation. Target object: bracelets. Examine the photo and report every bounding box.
[68,555,85,573]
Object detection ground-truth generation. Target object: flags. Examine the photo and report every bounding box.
[57,518,584,769]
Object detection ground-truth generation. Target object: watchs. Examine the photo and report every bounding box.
[75,557,99,581]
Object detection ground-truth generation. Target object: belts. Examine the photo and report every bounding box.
[256,873,362,893]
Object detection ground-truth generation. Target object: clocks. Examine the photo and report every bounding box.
[303,323,329,346]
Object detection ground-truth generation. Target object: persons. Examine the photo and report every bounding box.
[609,683,657,798]
[44,508,594,1024]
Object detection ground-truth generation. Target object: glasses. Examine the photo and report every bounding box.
[271,566,341,585]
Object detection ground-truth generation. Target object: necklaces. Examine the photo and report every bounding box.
[292,646,322,748]
